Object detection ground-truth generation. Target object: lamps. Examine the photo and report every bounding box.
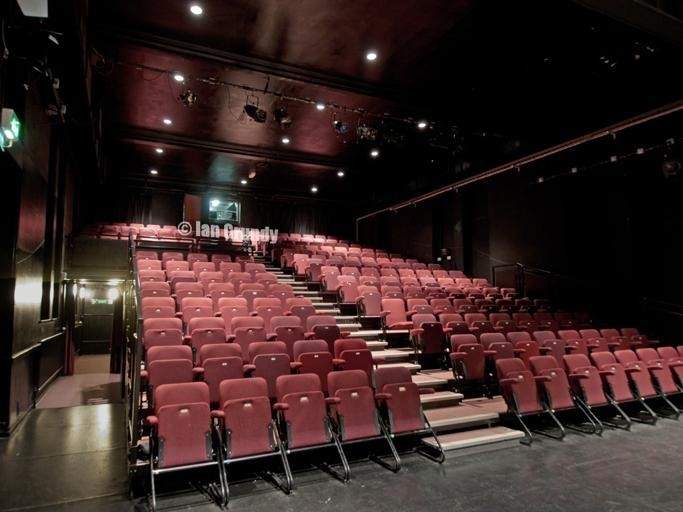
[245,89,266,123]
[0,108,21,149]
[274,95,291,127]
[330,106,350,134]
[357,115,376,141]
[178,80,197,109]
